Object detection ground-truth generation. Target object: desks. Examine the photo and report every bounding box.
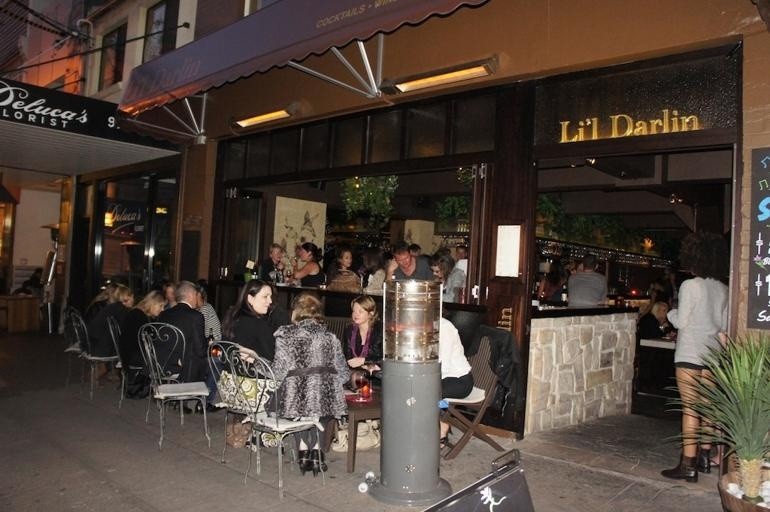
[635,338,680,399]
[347,389,382,473]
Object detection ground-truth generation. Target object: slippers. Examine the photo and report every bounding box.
[709,456,720,467]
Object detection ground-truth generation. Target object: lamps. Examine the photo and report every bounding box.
[378,56,498,95]
[227,108,291,130]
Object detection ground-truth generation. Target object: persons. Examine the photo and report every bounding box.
[567,254,608,308]
[359,239,470,303]
[220,279,275,446]
[661,244,729,482]
[660,326,664,330]
[82,281,222,413]
[326,248,362,289]
[258,244,285,283]
[636,302,668,365]
[290,243,324,288]
[30,268,43,288]
[240,290,350,477]
[439,318,473,449]
[343,296,383,398]
[552,261,583,301]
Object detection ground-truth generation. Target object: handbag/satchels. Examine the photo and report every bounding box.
[330,416,381,452]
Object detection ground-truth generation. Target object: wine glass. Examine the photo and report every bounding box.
[355,371,367,402]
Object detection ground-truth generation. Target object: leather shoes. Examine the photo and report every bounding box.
[698,459,710,474]
[661,468,698,483]
[196,404,214,412]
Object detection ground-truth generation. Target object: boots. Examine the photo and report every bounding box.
[311,449,328,477]
[298,450,311,476]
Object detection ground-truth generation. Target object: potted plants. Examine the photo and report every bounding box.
[663,327,770,512]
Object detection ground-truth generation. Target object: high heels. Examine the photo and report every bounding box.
[439,423,453,449]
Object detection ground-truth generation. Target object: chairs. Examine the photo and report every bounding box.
[230,350,326,498]
[62,311,271,463]
[440,323,517,460]
[322,297,352,353]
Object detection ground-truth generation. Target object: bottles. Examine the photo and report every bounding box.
[252,265,260,279]
[286,256,301,268]
[206,327,215,346]
[273,269,278,282]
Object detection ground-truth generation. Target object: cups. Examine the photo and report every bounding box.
[615,296,624,308]
